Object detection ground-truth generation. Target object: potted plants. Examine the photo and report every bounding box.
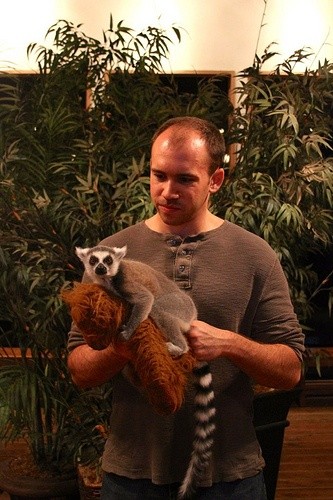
[0,10,333,500]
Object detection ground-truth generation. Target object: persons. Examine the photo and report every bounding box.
[66,115,308,498]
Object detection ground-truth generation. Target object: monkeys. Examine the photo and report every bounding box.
[74,244,218,500]
